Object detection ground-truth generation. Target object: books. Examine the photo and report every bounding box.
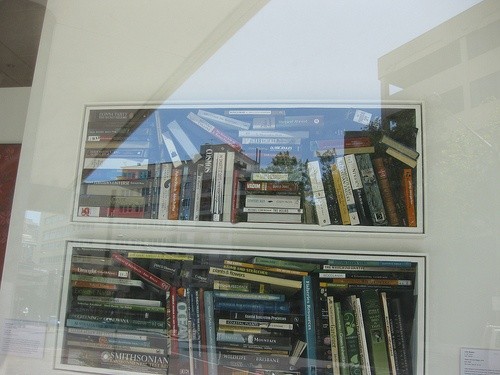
[59,245,419,375]
[79,105,420,229]
